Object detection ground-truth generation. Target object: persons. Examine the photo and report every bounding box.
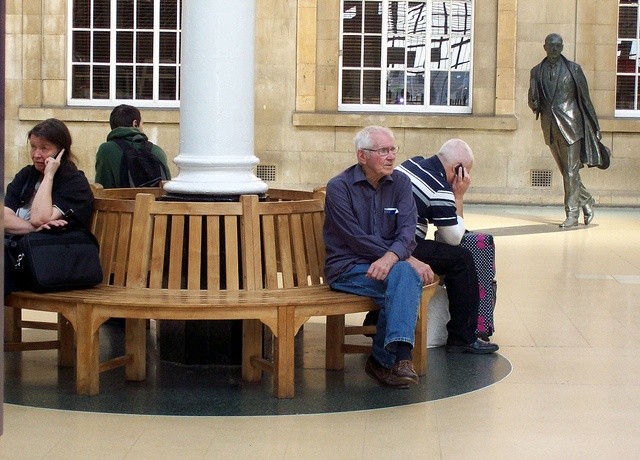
[362,138,501,357]
[2,117,96,305]
[322,123,435,390]
[95,104,171,191]
[527,32,611,228]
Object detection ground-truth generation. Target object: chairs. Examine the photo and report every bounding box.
[314,187,441,378]
[90,180,172,202]
[4,179,144,395]
[263,183,327,332]
[81,194,288,404]
[251,189,432,400]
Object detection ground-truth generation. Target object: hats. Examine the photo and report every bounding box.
[596,140,612,171]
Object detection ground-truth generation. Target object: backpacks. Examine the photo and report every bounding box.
[110,136,169,188]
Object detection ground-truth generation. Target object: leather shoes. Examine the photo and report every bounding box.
[365,356,411,390]
[582,198,595,225]
[445,340,500,355]
[394,360,420,385]
[559,216,579,228]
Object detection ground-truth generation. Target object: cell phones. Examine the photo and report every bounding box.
[54,147,70,163]
[453,162,467,178]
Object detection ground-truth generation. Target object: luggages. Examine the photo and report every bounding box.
[432,229,497,339]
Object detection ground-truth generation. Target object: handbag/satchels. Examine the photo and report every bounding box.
[26,208,103,293]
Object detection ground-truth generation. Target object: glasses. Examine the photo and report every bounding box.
[360,146,400,156]
